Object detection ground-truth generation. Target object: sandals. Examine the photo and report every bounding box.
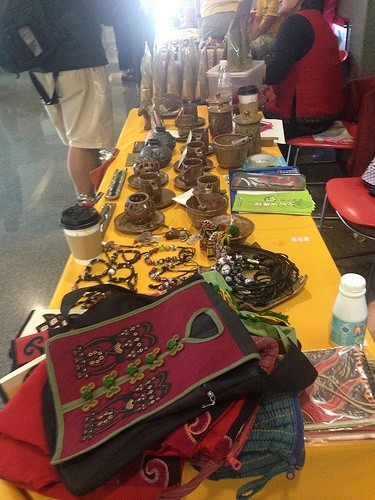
[121,73,137,82]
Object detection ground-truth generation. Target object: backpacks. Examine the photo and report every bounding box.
[0,0,59,73]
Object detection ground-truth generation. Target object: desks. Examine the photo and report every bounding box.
[0,105,375,500]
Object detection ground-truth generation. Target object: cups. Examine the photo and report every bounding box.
[138,172,162,203]
[179,158,204,186]
[59,206,102,265]
[187,141,206,166]
[183,103,198,121]
[237,85,259,114]
[205,92,234,138]
[191,128,209,148]
[197,175,220,195]
[233,112,263,153]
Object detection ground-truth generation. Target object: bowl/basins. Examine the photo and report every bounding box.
[207,214,255,246]
[213,133,251,169]
[185,194,228,230]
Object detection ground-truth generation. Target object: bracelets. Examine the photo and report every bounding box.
[72,239,199,310]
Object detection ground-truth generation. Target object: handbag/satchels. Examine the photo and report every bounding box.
[0,271,319,500]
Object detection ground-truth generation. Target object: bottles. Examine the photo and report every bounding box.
[329,273,368,348]
[217,60,232,106]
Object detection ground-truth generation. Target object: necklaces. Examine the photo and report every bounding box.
[213,243,298,305]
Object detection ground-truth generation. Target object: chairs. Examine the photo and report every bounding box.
[319,177,375,295]
[285,76,375,220]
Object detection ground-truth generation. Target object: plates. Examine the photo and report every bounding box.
[127,171,168,188]
[174,172,213,190]
[156,188,176,209]
[114,210,165,234]
[180,143,214,156]
[175,117,205,128]
[173,157,213,172]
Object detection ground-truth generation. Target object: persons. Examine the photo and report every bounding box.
[27,0,115,201]
[197,0,346,160]
[112,0,145,81]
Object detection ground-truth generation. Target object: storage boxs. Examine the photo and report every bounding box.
[207,60,267,97]
